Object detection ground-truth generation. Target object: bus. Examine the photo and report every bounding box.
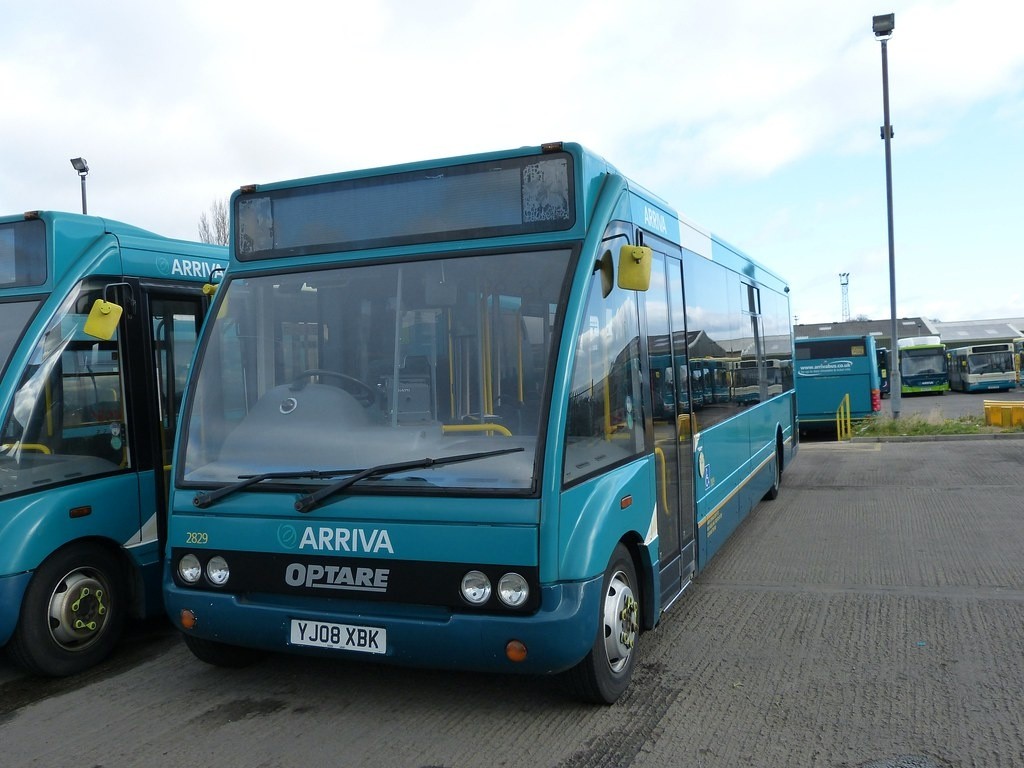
[888,336,951,397]
[945,337,1024,394]
[160,140,799,706]
[794,335,890,422]
[619,353,793,419]
[1,211,322,681]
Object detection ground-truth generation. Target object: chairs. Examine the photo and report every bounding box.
[526,390,541,434]
[398,356,449,417]
[78,400,121,461]
[493,394,521,435]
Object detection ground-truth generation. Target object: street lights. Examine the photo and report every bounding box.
[871,12,902,419]
[70,157,90,217]
